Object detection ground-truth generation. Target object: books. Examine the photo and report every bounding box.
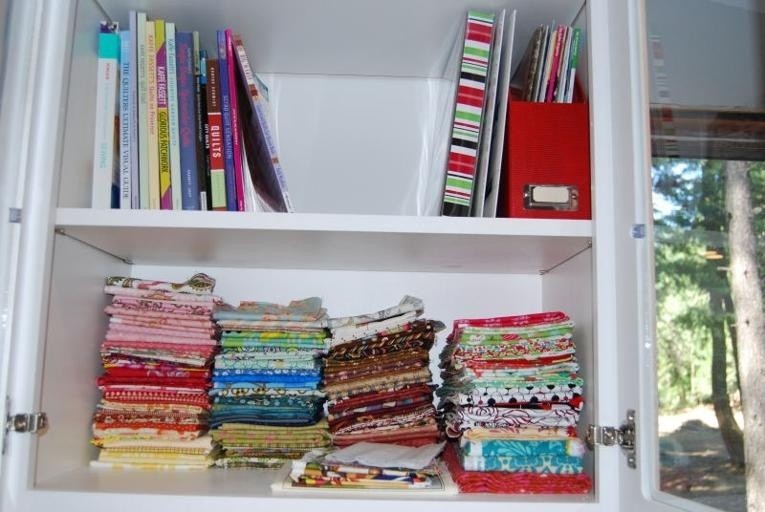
[421,8,581,216]
[91,10,294,213]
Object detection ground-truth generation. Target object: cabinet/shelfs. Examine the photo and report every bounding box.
[1,1,659,511]
